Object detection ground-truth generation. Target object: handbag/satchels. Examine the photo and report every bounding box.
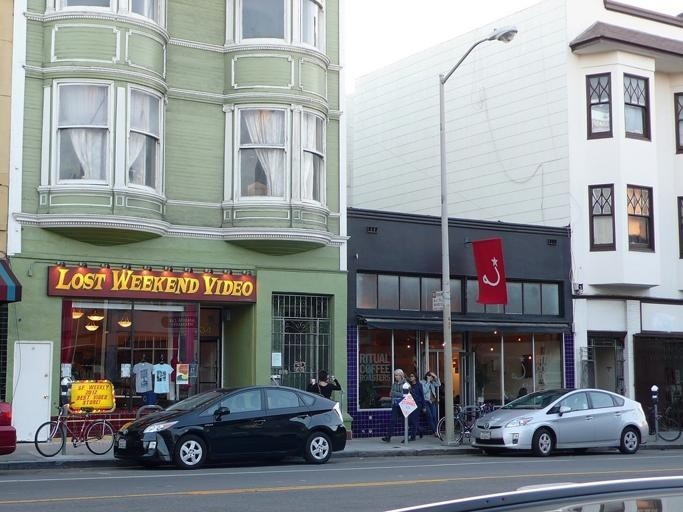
[430,390,439,405]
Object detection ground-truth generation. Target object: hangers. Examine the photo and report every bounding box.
[158,357,166,365]
[140,356,147,363]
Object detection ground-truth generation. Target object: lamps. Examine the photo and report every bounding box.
[86,309,104,321]
[55,260,252,277]
[117,310,133,328]
[84,320,100,332]
[71,308,84,320]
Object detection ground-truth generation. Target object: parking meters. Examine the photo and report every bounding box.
[649,383,658,442]
[403,381,411,446]
[60,375,72,455]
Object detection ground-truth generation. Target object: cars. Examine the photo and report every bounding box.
[0,398,18,456]
[111,385,351,470]
[468,387,651,459]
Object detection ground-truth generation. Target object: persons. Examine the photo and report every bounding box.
[379,369,411,444]
[407,372,425,441]
[307,371,341,400]
[419,372,441,438]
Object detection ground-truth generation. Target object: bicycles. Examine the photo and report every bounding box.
[435,401,488,444]
[644,401,682,443]
[33,400,116,459]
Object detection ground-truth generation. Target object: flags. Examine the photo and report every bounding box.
[472,236,507,305]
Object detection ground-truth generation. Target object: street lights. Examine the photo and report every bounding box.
[437,23,519,448]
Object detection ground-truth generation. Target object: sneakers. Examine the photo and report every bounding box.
[382,437,391,442]
[400,430,438,444]
